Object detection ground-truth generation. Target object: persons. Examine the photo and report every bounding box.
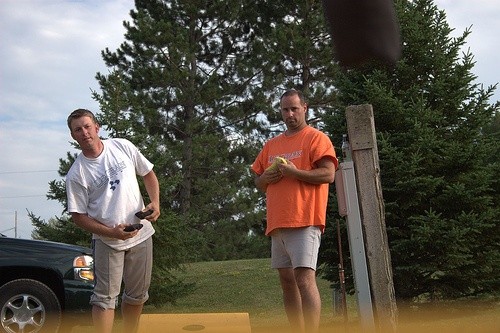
[251,90,339,333]
[66,108,160,333]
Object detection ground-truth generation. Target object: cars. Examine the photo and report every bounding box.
[0,236,96,333]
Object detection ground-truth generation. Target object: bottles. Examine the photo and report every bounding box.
[342,135,351,161]
